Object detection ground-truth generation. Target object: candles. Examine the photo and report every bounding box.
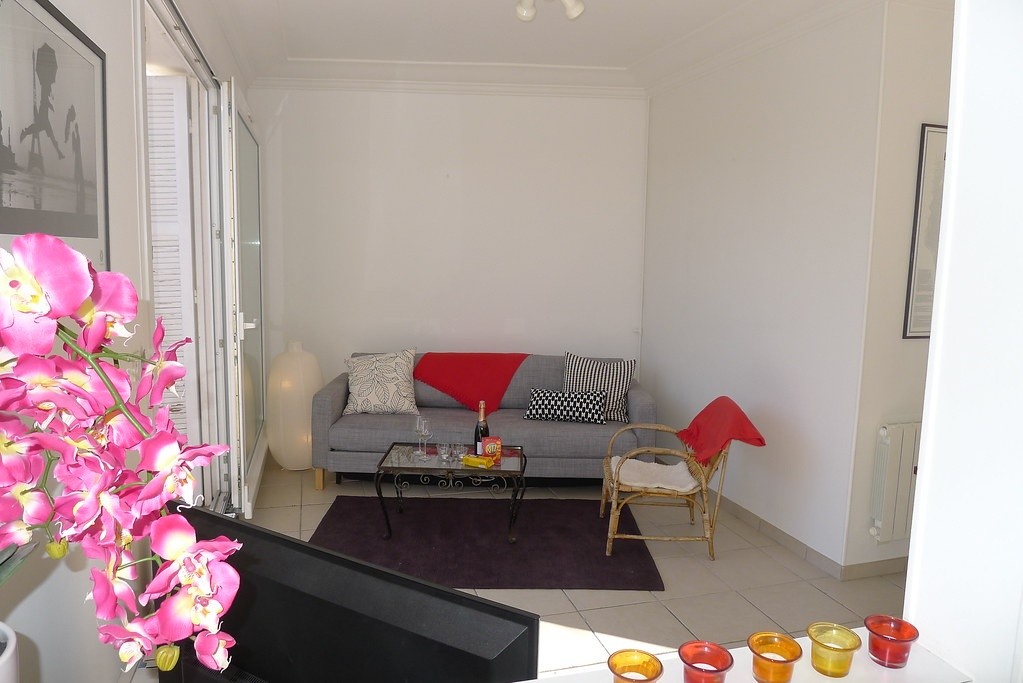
[864,614,919,669]
[807,621,863,679]
[608,649,664,683]
[747,630,804,683]
[678,640,735,683]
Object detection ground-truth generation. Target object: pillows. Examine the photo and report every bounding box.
[524,388,608,424]
[558,351,638,423]
[341,346,420,417]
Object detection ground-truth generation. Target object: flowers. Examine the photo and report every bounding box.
[0,234,244,675]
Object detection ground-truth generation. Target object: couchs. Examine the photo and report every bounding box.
[311,352,657,492]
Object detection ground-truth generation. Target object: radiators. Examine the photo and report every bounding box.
[869,422,923,542]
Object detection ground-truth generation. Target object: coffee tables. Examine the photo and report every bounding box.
[374,441,528,540]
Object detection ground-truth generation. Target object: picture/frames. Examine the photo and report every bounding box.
[0,0,111,272]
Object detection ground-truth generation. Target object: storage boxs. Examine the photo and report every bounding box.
[483,435,502,466]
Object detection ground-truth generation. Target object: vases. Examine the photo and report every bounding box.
[0,622,19,683]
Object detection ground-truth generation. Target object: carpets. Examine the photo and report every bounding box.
[308,494,665,592]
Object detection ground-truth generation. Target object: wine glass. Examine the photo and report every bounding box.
[413,415,426,456]
[419,419,434,461]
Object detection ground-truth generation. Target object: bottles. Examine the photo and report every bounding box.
[474,401,489,456]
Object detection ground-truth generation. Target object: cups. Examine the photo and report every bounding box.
[459,447,469,458]
[451,442,464,464]
[747,631,803,683]
[437,443,449,463]
[864,614,919,669]
[678,640,734,683]
[807,622,862,678]
[441,448,451,458]
[608,649,664,683]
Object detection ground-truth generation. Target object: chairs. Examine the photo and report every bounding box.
[598,396,767,562]
